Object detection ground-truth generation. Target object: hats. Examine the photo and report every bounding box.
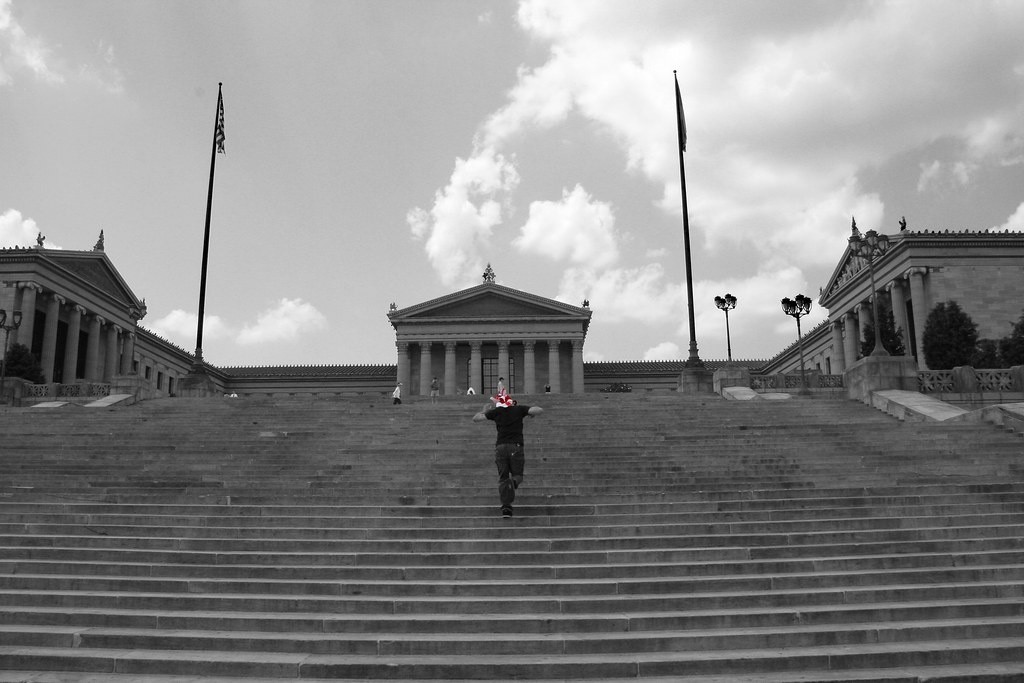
[398,382,403,385]
[490,389,517,408]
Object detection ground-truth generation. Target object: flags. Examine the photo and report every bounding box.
[675,74,687,152]
[214,82,226,156]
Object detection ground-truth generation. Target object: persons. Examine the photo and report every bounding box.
[544,382,551,394]
[392,383,403,405]
[430,377,440,406]
[498,377,504,394]
[472,388,543,518]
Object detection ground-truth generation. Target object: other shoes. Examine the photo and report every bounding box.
[508,478,515,501]
[502,510,512,517]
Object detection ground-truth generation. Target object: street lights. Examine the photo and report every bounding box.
[0,308,24,378]
[849,229,892,356]
[714,293,738,368]
[780,294,813,395]
